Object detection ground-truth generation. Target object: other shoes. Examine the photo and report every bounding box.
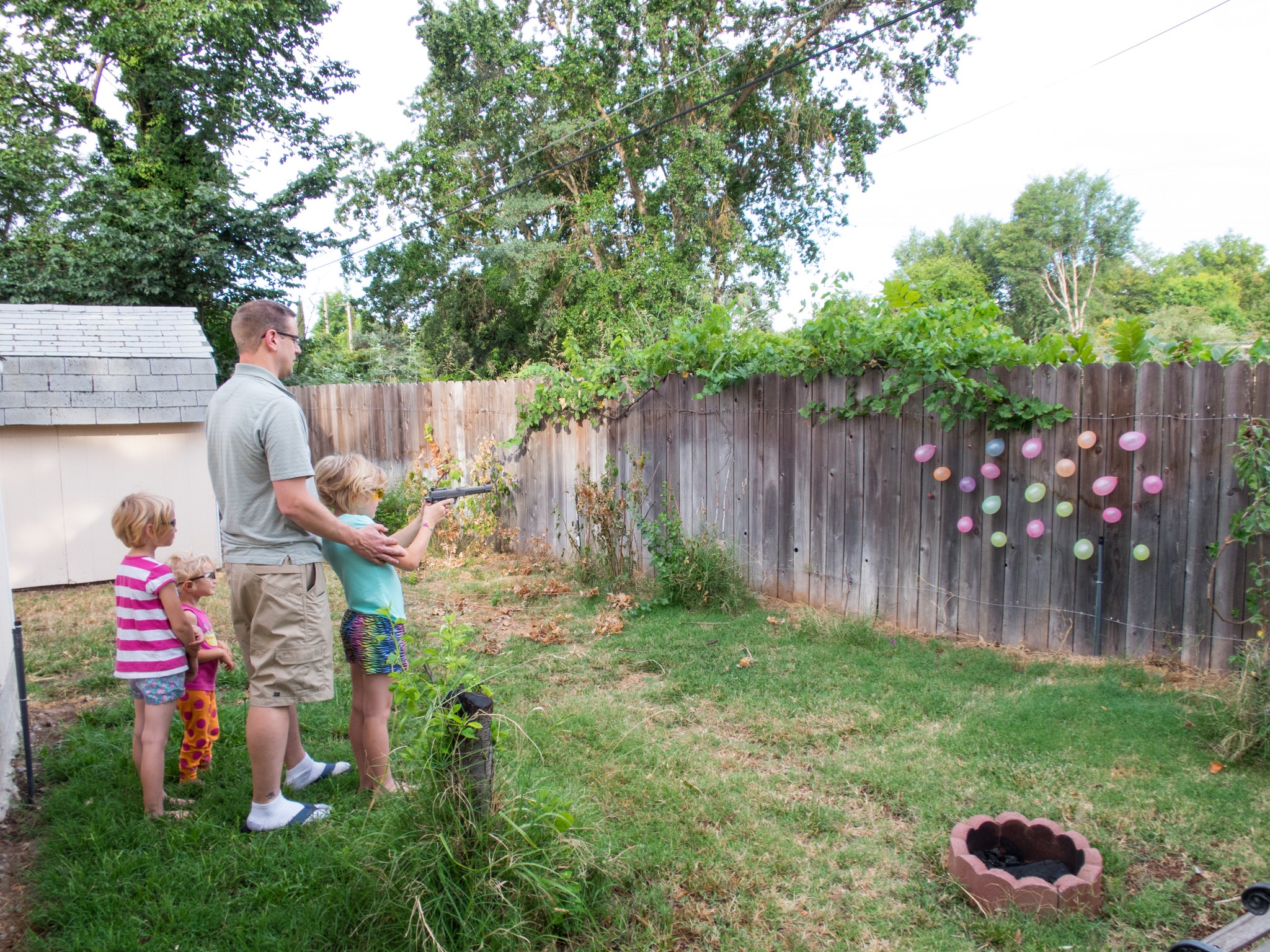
[166,811,190,821]
[166,797,197,807]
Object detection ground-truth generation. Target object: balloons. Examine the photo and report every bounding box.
[986,438,1004,456]
[1056,501,1073,517]
[958,517,973,533]
[1025,483,1046,503]
[960,477,976,492]
[1078,431,1096,449]
[914,444,935,462]
[1027,520,1044,538]
[982,495,1001,515]
[981,463,1000,479]
[1022,438,1042,458]
[991,531,1007,547]
[1143,475,1163,493]
[1133,544,1150,561]
[934,467,951,481]
[1119,431,1146,451]
[1074,538,1094,560]
[1102,507,1122,523]
[1056,459,1076,477]
[1093,476,1117,496]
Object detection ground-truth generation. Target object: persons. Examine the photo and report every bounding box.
[314,453,452,798]
[164,551,234,786]
[205,300,406,835]
[111,492,204,821]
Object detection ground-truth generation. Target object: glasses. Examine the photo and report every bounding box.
[176,572,216,586]
[160,519,176,527]
[262,330,301,346]
[370,488,384,500]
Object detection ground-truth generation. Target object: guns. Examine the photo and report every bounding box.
[426,484,492,504]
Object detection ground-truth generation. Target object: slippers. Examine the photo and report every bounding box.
[299,762,354,790]
[240,802,332,836]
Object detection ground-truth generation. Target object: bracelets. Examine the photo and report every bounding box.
[420,523,433,532]
